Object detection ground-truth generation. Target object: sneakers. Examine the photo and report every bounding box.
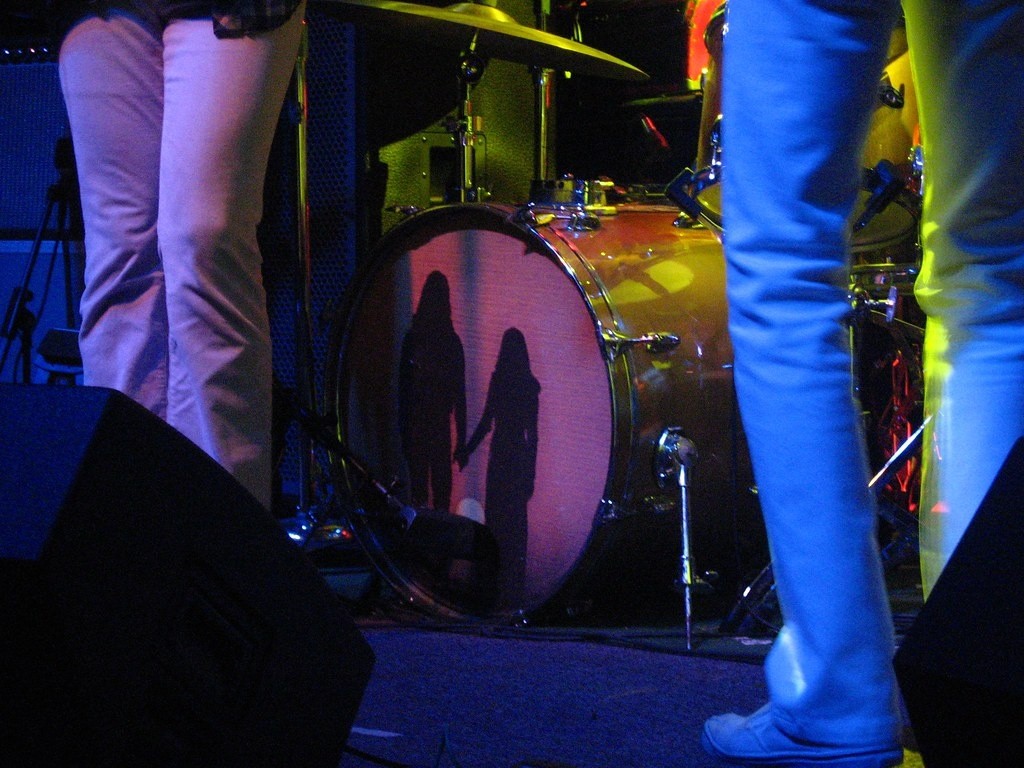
[701,703,905,767]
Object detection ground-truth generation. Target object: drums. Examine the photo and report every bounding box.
[685,0,923,257]
[324,192,776,635]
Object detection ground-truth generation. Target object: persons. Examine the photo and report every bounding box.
[40,0,307,516]
[700,0,1024,768]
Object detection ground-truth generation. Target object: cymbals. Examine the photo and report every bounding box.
[326,0,651,81]
